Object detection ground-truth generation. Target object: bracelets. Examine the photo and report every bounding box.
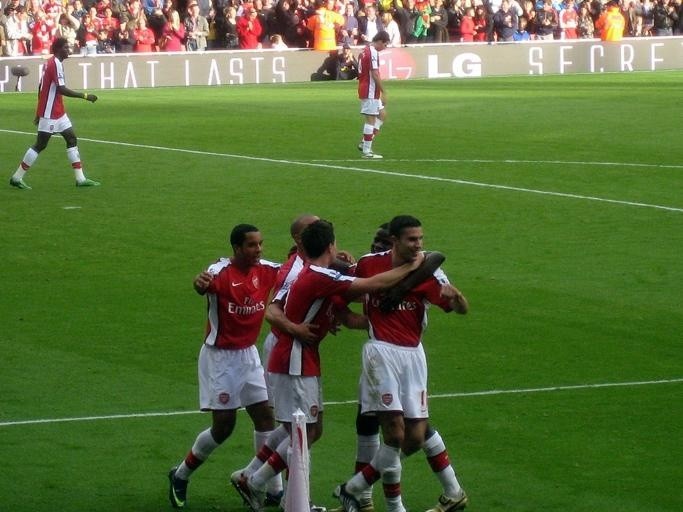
[83,92,88,99]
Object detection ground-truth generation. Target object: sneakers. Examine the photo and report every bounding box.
[75,178,101,186]
[231,470,467,511]
[168,467,188,509]
[9,176,31,190]
[361,152,383,159]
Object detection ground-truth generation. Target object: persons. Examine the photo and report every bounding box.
[230,213,470,512]
[357,29,388,161]
[9,35,105,191]
[0,1,683,80]
[168,223,283,512]
